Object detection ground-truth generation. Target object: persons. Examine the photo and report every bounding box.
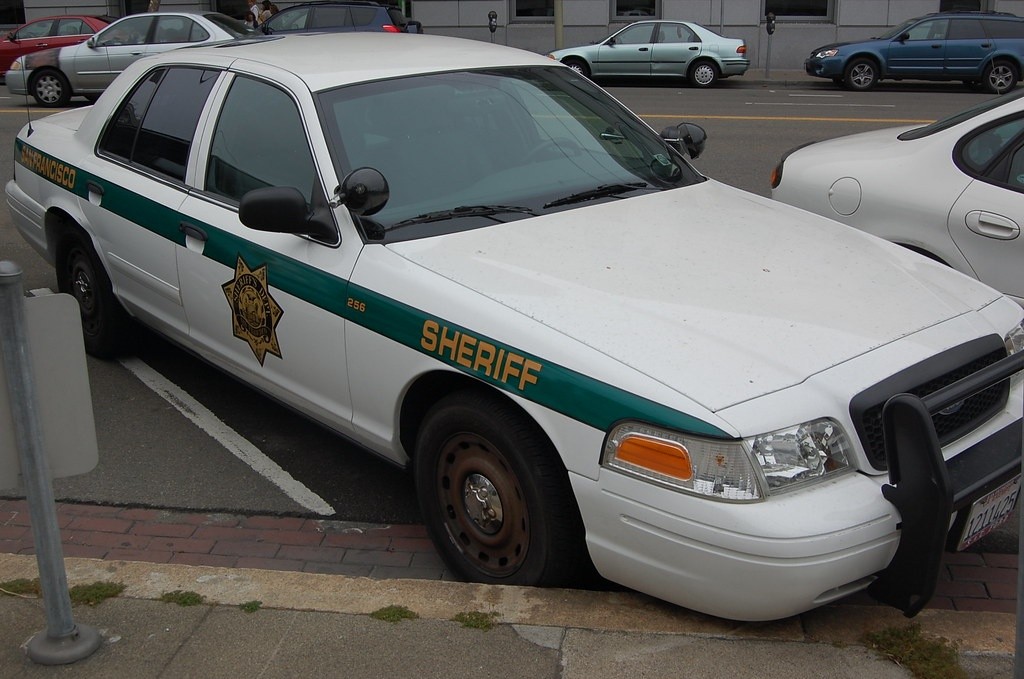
[243,0,280,29]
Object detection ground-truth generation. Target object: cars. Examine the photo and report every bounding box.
[5,8,263,108]
[766,85,1024,311]
[0,13,141,78]
[541,18,751,88]
[0,31,1024,627]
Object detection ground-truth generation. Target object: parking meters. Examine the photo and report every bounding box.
[487,11,498,44]
[765,12,777,79]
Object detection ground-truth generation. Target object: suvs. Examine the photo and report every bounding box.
[254,2,410,36]
[803,8,1024,94]
